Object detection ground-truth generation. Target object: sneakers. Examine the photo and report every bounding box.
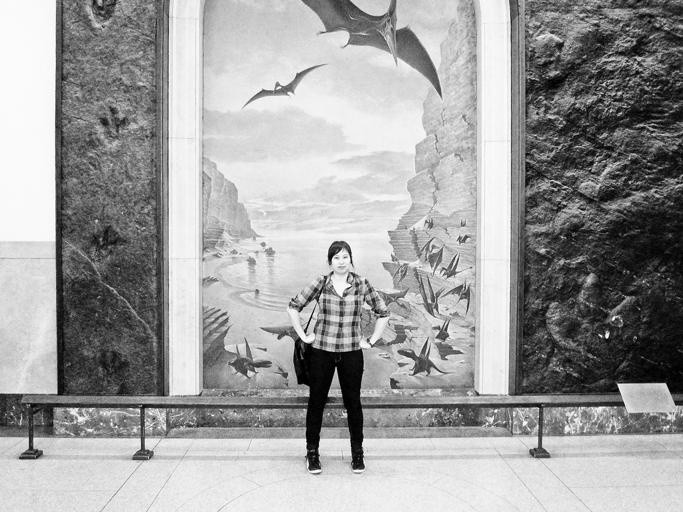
[304,449,322,474]
[350,449,365,473]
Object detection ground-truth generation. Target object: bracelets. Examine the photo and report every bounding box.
[367,339,373,347]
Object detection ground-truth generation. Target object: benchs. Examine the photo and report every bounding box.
[17,392,683,459]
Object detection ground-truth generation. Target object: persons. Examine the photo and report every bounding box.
[288,240,391,474]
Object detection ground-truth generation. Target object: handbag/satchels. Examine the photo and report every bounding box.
[293,337,310,386]
